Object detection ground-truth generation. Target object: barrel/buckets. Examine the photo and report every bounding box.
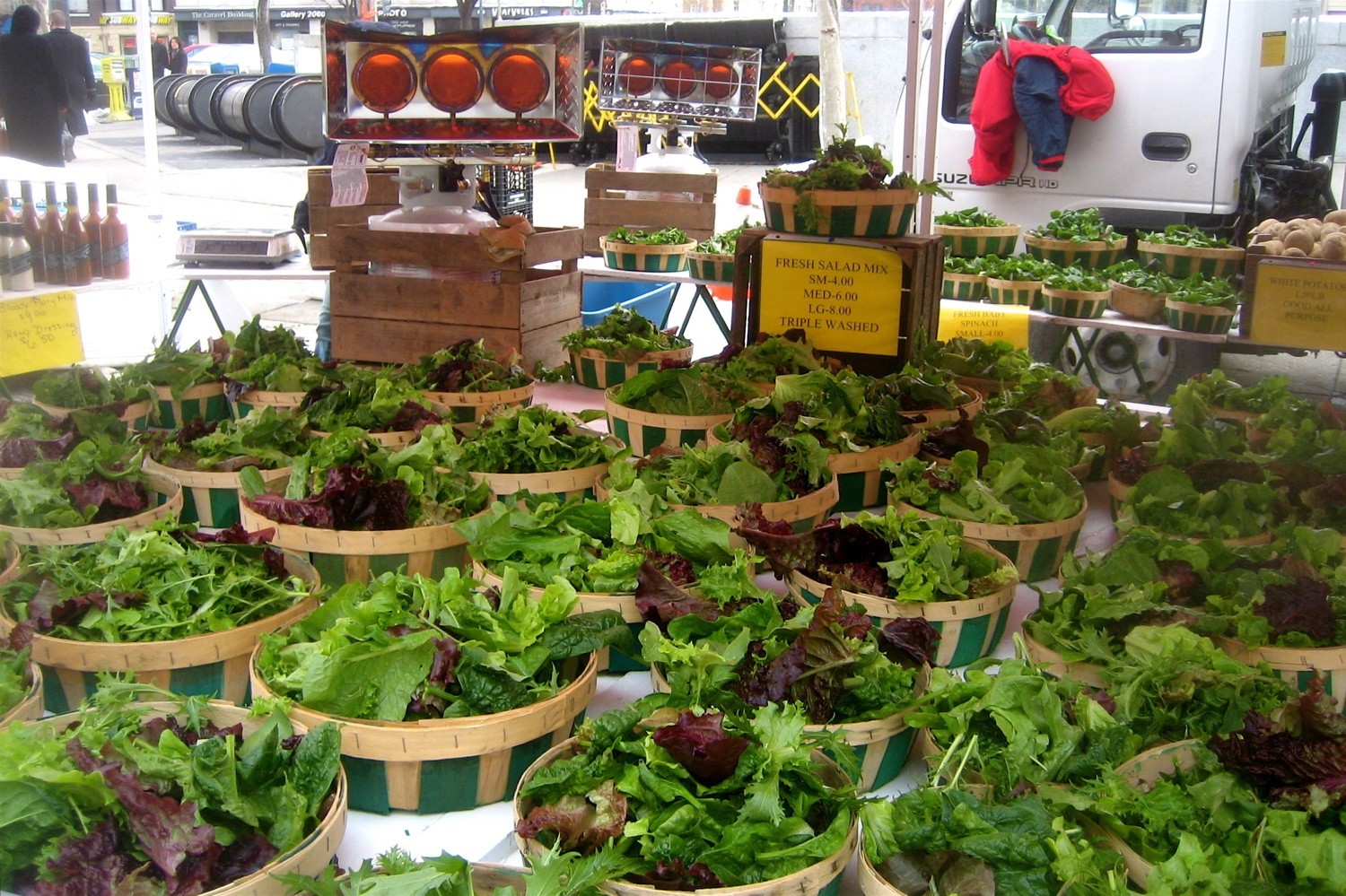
[579,277,677,338]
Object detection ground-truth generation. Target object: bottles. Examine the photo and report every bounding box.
[0,179,130,292]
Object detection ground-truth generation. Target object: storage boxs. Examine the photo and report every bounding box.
[327,223,586,376]
[583,162,717,257]
[1239,227,1346,339]
[306,169,404,270]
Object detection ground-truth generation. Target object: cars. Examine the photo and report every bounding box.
[181,42,293,76]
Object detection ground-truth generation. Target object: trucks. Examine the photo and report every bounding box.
[898,1,1346,406]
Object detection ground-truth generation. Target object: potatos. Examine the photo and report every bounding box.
[1254,209,1346,263]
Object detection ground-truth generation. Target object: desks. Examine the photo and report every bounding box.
[0,276,144,304]
[163,252,748,348]
[941,298,1229,407]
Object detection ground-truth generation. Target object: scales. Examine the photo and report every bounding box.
[175,227,302,268]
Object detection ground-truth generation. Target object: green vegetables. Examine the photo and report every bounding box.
[761,122,955,232]
[0,309,1346,896]
[932,204,1242,313]
[607,215,762,254]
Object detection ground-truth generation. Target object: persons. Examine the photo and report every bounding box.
[164,36,187,74]
[0,4,68,167]
[150,29,167,84]
[41,10,97,163]
[291,20,427,367]
